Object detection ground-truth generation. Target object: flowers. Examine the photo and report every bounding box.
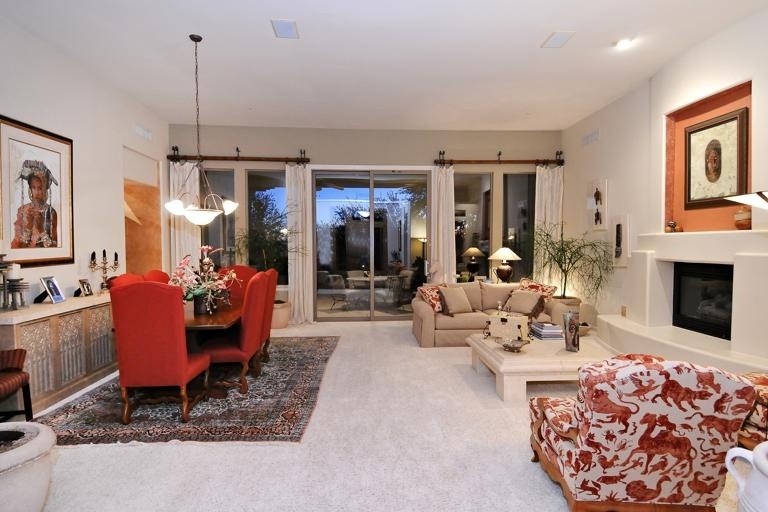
[168,245,244,308]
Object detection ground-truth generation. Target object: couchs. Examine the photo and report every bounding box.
[411,281,573,349]
[526,352,759,511]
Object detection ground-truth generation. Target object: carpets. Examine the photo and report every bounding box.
[29,336,340,445]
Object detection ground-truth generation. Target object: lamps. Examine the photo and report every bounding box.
[487,246,522,282]
[461,246,484,273]
[163,162,239,228]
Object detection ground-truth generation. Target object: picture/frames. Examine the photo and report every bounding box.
[33,274,66,304]
[74,277,93,297]
[684,106,748,210]
[1,116,76,270]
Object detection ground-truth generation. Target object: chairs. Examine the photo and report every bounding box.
[1,347,34,422]
[205,270,270,396]
[112,270,143,289]
[346,270,364,278]
[97,284,213,422]
[143,270,170,283]
[217,265,258,299]
[399,270,414,304]
[260,267,278,362]
[328,273,346,311]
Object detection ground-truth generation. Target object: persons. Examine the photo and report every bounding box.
[10,160,58,249]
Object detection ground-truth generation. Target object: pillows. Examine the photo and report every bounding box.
[437,285,474,313]
[417,281,449,312]
[518,277,558,304]
[503,288,542,316]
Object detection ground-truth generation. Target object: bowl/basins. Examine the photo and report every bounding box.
[578,321,592,336]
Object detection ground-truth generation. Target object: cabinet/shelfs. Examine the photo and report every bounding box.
[0,293,119,416]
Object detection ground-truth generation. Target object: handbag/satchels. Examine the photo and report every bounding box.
[483,314,530,346]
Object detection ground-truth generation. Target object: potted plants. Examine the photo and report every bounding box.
[534,222,614,325]
[231,190,309,329]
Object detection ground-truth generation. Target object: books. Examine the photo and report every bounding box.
[529,321,565,340]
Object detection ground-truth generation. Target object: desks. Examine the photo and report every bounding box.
[347,275,388,288]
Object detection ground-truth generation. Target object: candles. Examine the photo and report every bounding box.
[114,252,118,261]
[7,262,22,279]
[91,250,95,261]
[102,248,106,257]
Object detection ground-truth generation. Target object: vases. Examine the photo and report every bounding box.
[193,296,210,315]
